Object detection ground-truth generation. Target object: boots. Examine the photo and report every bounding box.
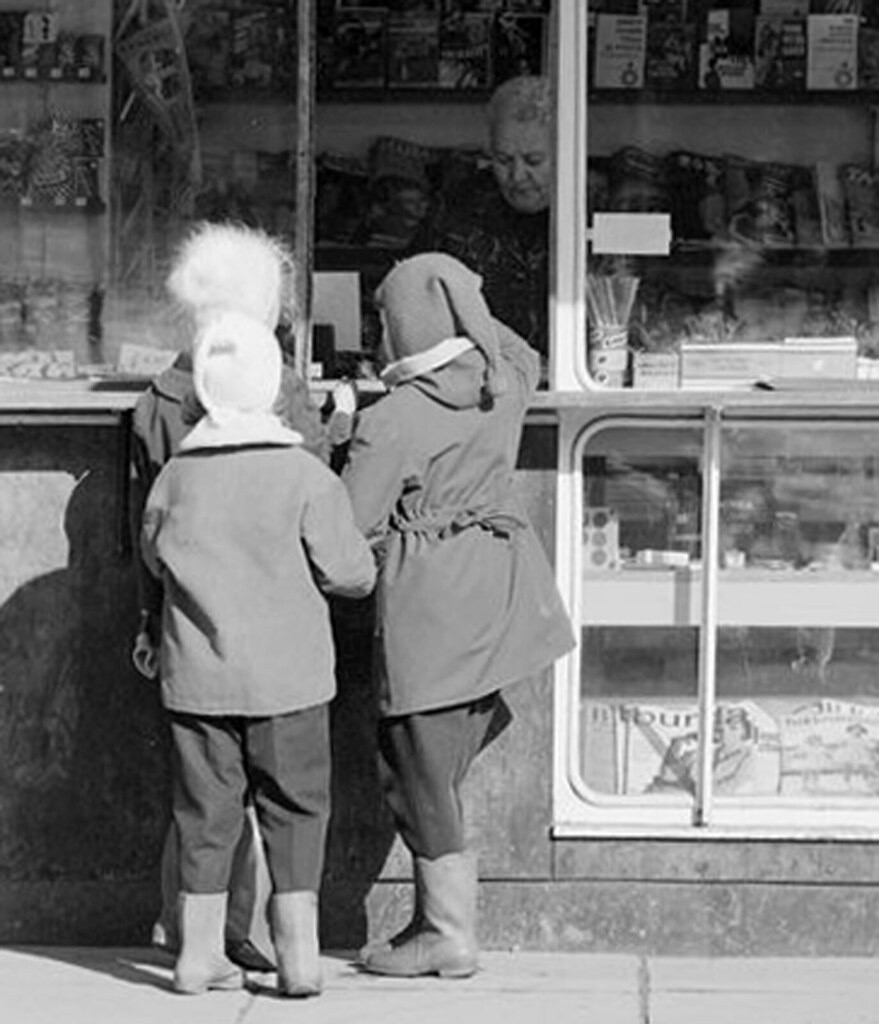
[151,820,180,955]
[357,852,479,977]
[174,891,247,993]
[226,807,278,970]
[272,891,322,994]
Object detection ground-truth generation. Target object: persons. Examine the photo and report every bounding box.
[450,76,637,382]
[145,314,382,999]
[343,252,578,976]
[133,218,337,976]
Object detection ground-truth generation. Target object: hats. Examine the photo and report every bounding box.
[375,253,509,396]
[176,317,303,452]
